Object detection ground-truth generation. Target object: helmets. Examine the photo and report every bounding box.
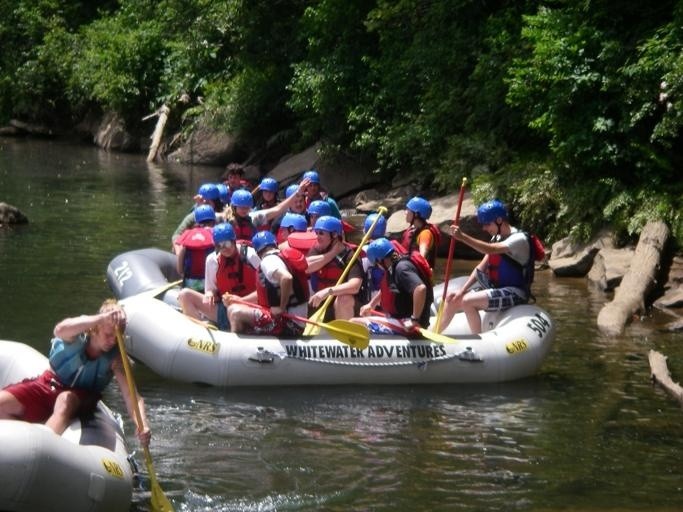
[252,229,278,254]
[212,222,237,242]
[194,169,343,209]
[365,236,395,265]
[477,199,508,225]
[192,204,218,222]
[363,213,387,238]
[306,200,333,217]
[312,214,342,237]
[406,195,433,221]
[280,212,308,233]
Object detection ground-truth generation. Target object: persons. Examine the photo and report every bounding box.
[0,300,151,446]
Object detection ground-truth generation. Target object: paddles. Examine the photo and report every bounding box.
[434,176,468,334]
[366,309,455,342]
[114,324,174,512]
[225,290,369,350]
[302,205,388,336]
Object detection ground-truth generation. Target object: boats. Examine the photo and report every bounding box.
[0,338,133,511]
[108,271,556,385]
[103,246,186,298]
